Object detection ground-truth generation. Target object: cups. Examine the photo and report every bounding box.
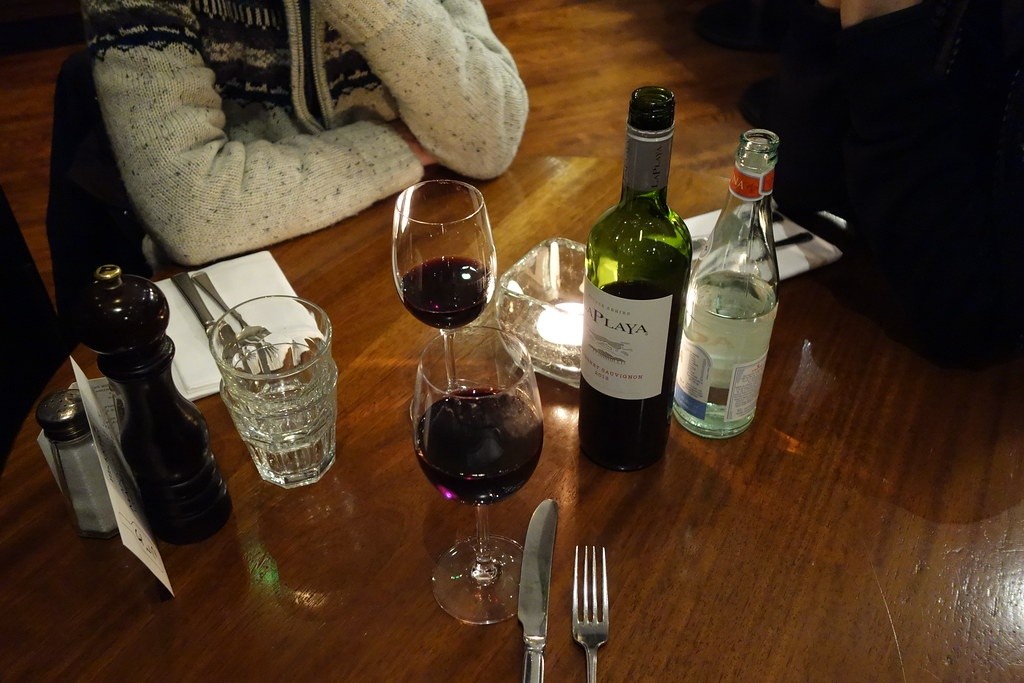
[498,237,587,388]
[208,295,340,488]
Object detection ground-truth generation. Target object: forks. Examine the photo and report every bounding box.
[572,545,610,683]
[192,271,272,346]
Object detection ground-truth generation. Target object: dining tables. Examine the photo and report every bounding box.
[0,0,1024,683]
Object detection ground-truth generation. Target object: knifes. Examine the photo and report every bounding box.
[692,211,785,253]
[170,270,238,353]
[518,499,558,683]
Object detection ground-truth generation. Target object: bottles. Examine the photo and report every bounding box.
[671,128,784,439]
[35,388,120,541]
[575,86,693,474]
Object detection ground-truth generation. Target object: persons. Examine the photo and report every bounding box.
[81,0,528,266]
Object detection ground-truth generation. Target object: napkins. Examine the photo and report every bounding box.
[682,208,843,281]
[154,251,326,402]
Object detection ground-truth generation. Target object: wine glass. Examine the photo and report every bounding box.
[412,325,544,625]
[390,179,498,388]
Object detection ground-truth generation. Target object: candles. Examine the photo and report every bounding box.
[496,236,586,385]
